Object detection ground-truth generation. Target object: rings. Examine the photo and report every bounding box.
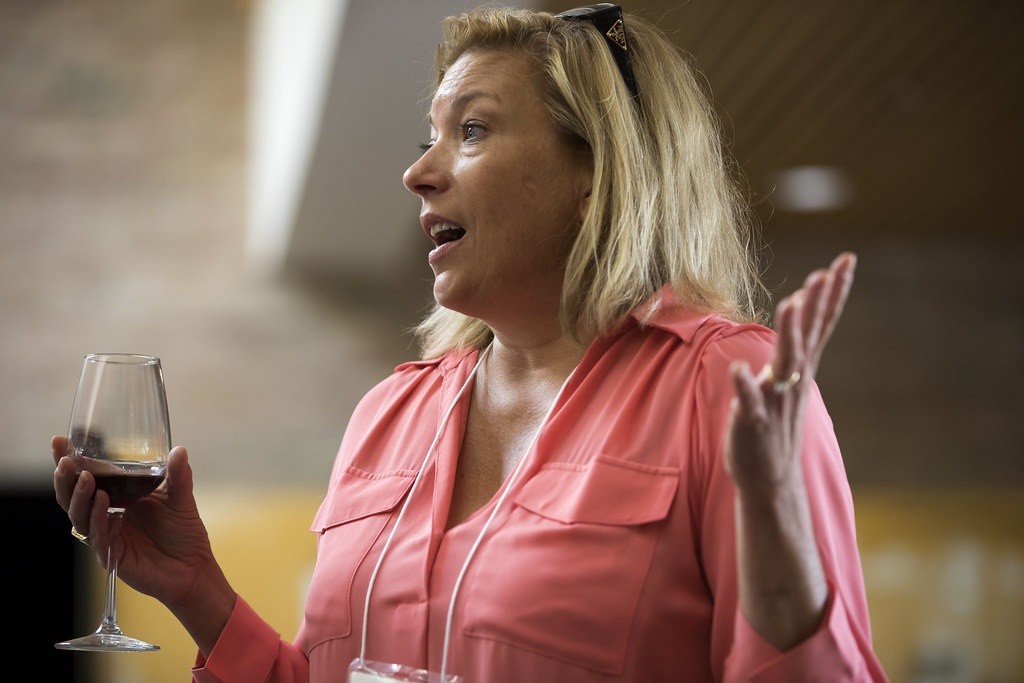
[71,526,91,547]
[763,360,800,396]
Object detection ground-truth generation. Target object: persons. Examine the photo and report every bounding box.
[50,5,890,683]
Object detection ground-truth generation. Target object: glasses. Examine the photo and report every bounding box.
[553,2,641,106]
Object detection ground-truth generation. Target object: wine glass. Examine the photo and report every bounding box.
[54,352,173,653]
